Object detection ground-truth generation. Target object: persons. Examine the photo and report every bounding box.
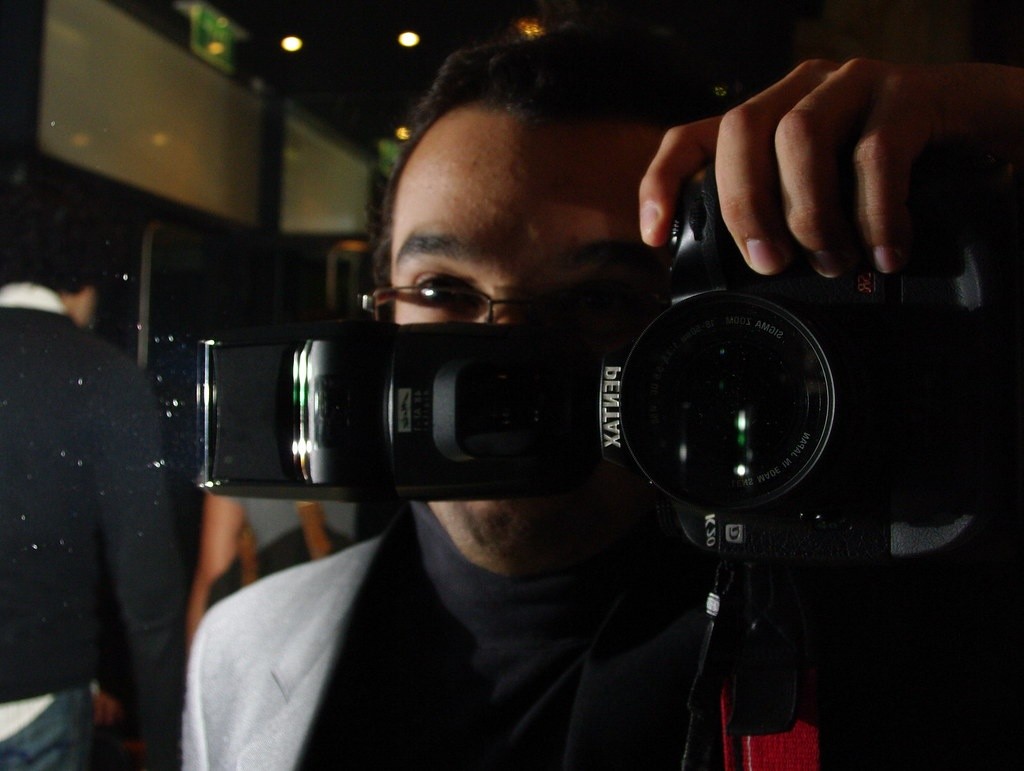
[0,215,355,771]
[175,22,1024,771]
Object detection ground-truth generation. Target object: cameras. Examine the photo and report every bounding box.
[196,144,1003,564]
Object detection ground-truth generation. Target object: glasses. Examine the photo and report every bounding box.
[362,282,647,338]
